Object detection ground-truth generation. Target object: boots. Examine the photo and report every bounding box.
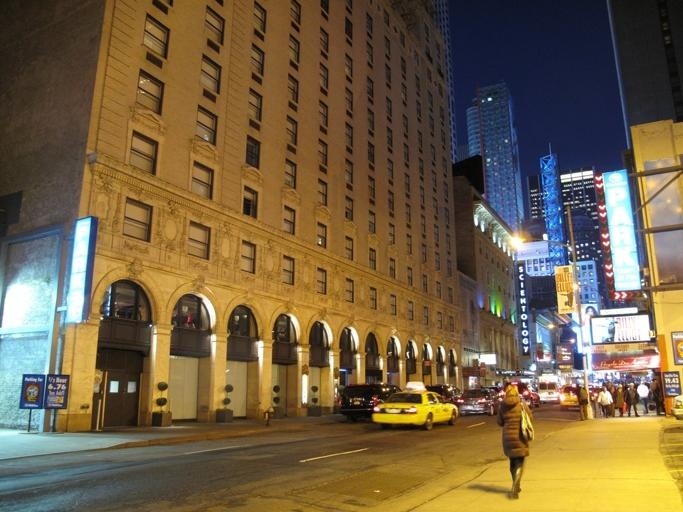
[507,464,523,499]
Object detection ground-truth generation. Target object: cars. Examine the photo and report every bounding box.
[670,394,683,421]
[337,378,604,433]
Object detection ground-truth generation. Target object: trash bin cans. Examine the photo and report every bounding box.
[580,398,588,420]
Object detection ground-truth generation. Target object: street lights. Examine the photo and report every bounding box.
[548,323,557,370]
[507,203,594,421]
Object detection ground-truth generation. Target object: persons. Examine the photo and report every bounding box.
[576,375,663,421]
[497,384,531,500]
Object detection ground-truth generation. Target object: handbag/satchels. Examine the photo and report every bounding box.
[520,403,534,441]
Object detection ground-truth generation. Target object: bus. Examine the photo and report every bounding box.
[536,373,566,404]
